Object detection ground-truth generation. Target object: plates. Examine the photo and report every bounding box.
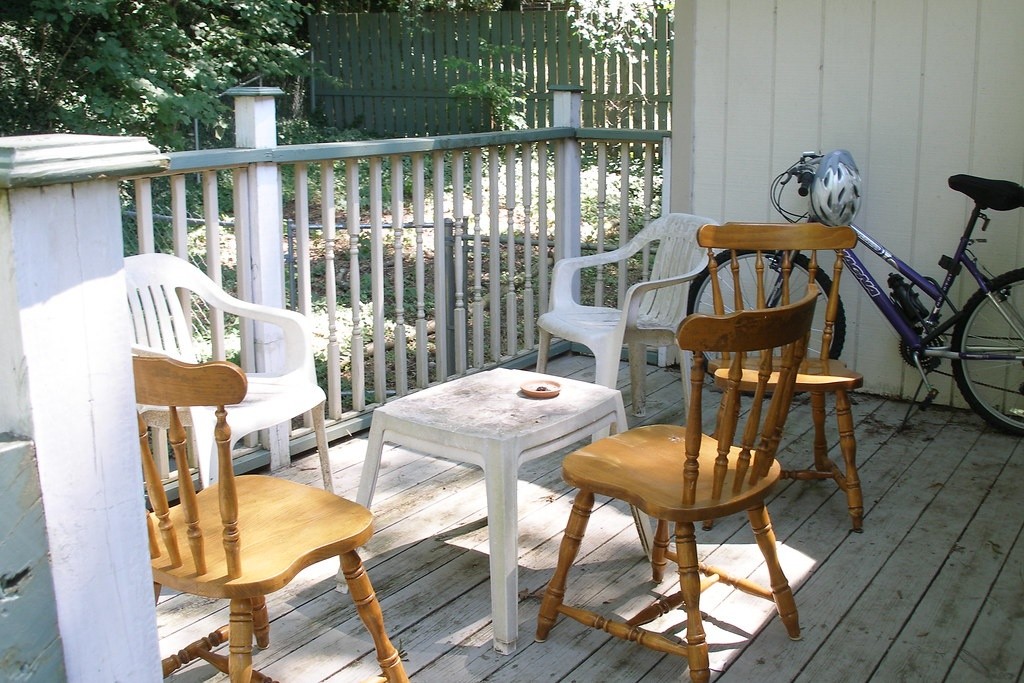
[520,380,561,398]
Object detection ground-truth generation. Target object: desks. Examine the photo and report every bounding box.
[333,368,654,655]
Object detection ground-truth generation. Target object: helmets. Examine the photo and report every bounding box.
[808,148,862,227]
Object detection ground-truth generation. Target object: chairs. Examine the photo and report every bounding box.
[538,213,721,443]
[696,222,866,534]
[131,357,409,683]
[535,283,820,683]
[123,253,333,494]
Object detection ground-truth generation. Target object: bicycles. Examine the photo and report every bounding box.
[686,146,1023,435]
